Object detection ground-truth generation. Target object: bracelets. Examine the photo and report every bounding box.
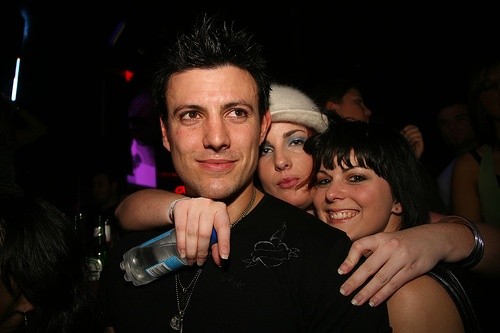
[435,214,484,269]
[168,197,192,223]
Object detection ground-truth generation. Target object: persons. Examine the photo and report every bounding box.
[96,14,393,333]
[313,62,500,229]
[114,85,500,308]
[0,174,122,333]
[304,118,500,333]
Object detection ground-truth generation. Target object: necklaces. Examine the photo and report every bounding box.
[170,185,257,333]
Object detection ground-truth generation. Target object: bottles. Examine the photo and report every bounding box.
[120,227,217,286]
[86,212,113,285]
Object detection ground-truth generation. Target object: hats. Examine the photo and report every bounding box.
[268,83,329,133]
